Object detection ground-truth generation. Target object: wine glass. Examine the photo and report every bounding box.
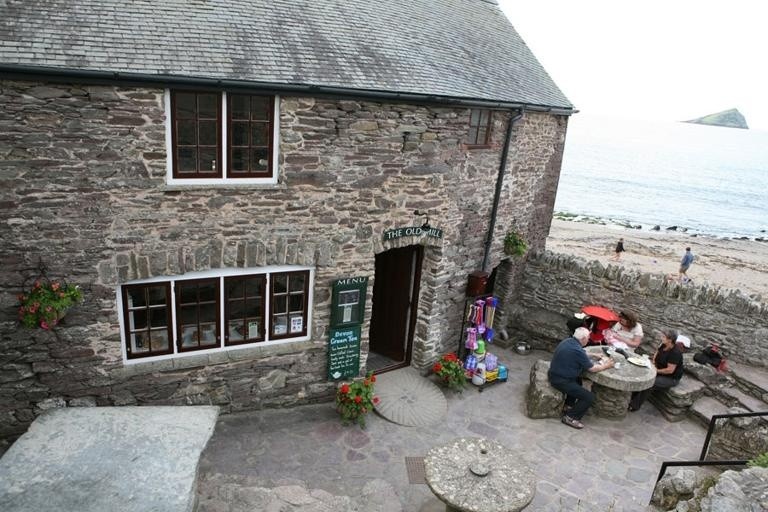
[607,346,616,359]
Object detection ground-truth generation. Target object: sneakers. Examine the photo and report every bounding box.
[562,405,584,428]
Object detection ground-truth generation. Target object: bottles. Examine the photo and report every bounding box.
[135,332,143,348]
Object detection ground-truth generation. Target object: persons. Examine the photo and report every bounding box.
[616,238,625,260]
[548,327,615,429]
[605,309,643,351]
[628,328,683,411]
[679,248,693,283]
[604,329,628,350]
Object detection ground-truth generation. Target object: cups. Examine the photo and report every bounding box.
[642,354,649,364]
[615,362,620,369]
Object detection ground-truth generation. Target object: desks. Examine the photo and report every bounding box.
[584,345,658,420]
[0,404,219,512]
[424,435,536,512]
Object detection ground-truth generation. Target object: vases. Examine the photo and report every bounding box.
[507,243,519,254]
[340,401,358,419]
[441,374,454,385]
[41,301,72,324]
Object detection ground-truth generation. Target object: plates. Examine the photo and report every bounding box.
[628,357,646,367]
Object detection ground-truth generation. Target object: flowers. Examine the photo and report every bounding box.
[432,353,463,378]
[505,225,526,249]
[20,277,81,325]
[338,375,380,413]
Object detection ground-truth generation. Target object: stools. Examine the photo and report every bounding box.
[528,359,566,418]
[651,370,705,423]
